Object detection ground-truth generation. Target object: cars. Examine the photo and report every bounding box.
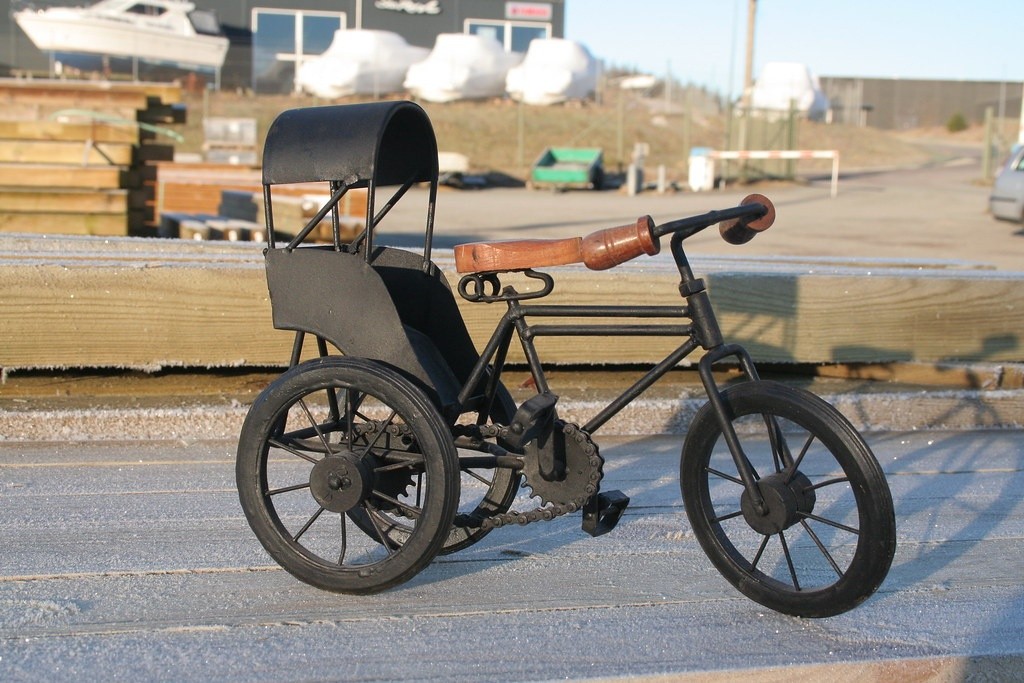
[988,142,1024,226]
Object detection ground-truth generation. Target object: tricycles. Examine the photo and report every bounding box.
[236,99,898,620]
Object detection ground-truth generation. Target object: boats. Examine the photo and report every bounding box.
[13,0,231,91]
